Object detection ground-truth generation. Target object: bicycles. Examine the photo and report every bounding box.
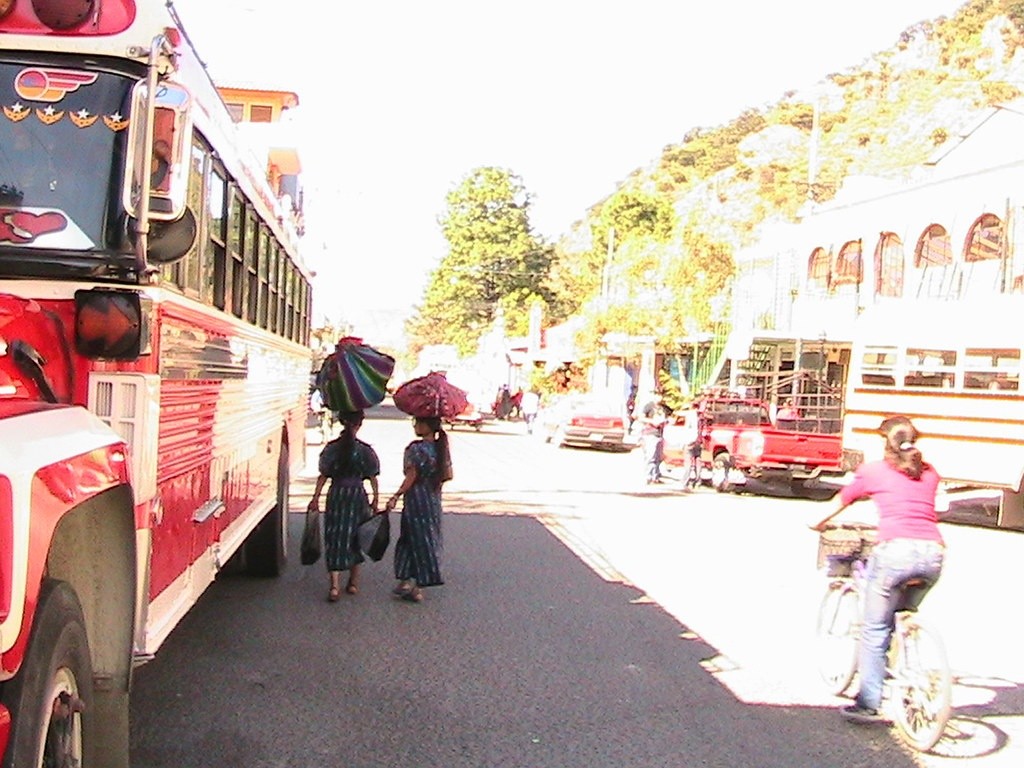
[809,519,956,753]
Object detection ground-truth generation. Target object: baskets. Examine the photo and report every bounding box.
[815,521,880,577]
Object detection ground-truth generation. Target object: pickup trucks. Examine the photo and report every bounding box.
[659,396,845,495]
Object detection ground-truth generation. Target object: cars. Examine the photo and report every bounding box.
[532,394,625,453]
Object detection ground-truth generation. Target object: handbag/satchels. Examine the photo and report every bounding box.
[350,508,391,563]
[300,507,321,564]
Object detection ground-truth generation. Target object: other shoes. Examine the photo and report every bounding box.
[841,704,881,722]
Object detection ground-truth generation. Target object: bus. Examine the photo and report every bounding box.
[0,0,315,768]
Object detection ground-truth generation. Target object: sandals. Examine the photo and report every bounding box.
[346,584,358,594]
[329,587,339,600]
[394,582,424,602]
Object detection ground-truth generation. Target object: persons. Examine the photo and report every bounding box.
[777,398,796,418]
[387,417,468,602]
[644,403,668,485]
[308,411,396,600]
[811,417,946,722]
[496,384,510,405]
[684,403,706,492]
